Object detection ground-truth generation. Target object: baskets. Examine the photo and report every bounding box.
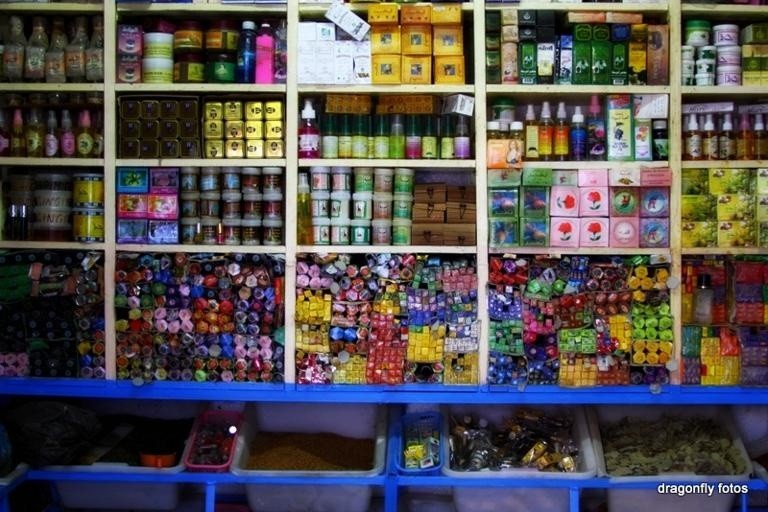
[183,407,244,474]
[393,410,444,477]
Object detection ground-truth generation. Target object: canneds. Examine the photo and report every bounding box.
[5,171,104,240]
[142,19,241,82]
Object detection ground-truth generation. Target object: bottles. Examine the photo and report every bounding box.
[236,20,255,83]
[682,110,767,161]
[297,173,314,244]
[0,9,105,158]
[692,272,714,324]
[255,23,275,83]
[487,94,668,160]
[298,96,472,159]
